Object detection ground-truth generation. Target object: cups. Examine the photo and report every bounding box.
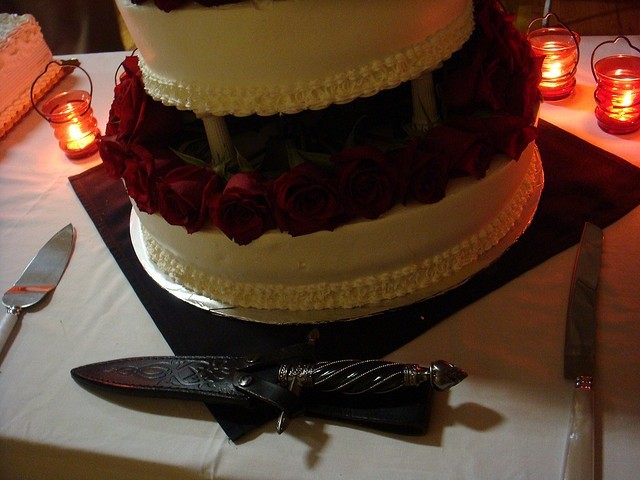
[595,54,639,135]
[527,28,578,103]
[44,91,102,158]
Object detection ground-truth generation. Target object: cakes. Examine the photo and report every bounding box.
[113,0,545,326]
[0,11,64,137]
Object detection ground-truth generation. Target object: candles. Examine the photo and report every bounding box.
[591,35,639,136]
[30,62,101,159]
[529,14,581,98]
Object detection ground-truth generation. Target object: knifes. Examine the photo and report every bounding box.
[564,219,603,480]
[1,223,76,359]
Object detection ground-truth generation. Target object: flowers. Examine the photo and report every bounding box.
[111,57,142,86]
[97,135,152,179]
[439,3,542,180]
[154,155,231,234]
[211,153,275,245]
[106,79,178,142]
[267,117,339,234]
[394,116,448,204]
[119,158,193,211]
[336,117,399,220]
